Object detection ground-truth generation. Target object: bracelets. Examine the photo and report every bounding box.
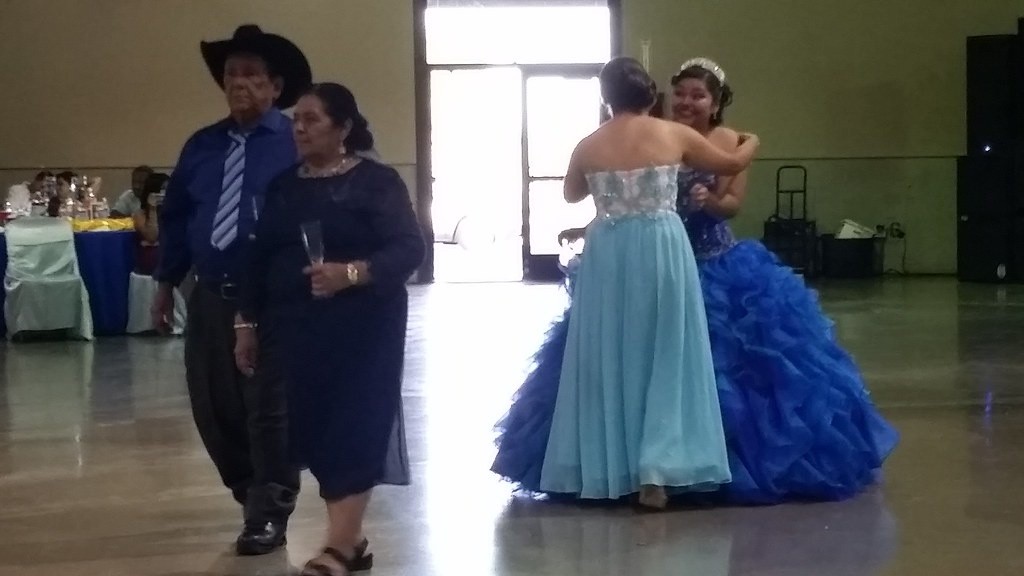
[233,324,257,329]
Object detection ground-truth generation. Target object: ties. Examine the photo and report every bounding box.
[210,129,254,250]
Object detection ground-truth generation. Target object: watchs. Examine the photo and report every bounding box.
[347,263,360,285]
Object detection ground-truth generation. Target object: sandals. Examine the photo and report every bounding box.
[301,537,373,576]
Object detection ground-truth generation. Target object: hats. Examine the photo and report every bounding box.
[680,57,726,88]
[201,24,312,109]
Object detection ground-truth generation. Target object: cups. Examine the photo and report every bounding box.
[300,224,324,264]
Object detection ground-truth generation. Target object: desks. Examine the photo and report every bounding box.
[0,229,139,337]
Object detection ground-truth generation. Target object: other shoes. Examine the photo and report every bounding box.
[639,485,668,511]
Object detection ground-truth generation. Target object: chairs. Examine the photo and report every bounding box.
[5,215,95,343]
[127,271,187,335]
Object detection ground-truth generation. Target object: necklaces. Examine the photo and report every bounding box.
[300,156,355,177]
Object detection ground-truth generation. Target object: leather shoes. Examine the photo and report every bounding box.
[237,517,287,556]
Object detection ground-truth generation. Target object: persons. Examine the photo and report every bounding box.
[221,83,426,576]
[490,54,899,504]
[5,164,171,277]
[148,22,310,558]
[538,57,759,508]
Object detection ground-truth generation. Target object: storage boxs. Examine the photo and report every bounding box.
[820,219,888,277]
